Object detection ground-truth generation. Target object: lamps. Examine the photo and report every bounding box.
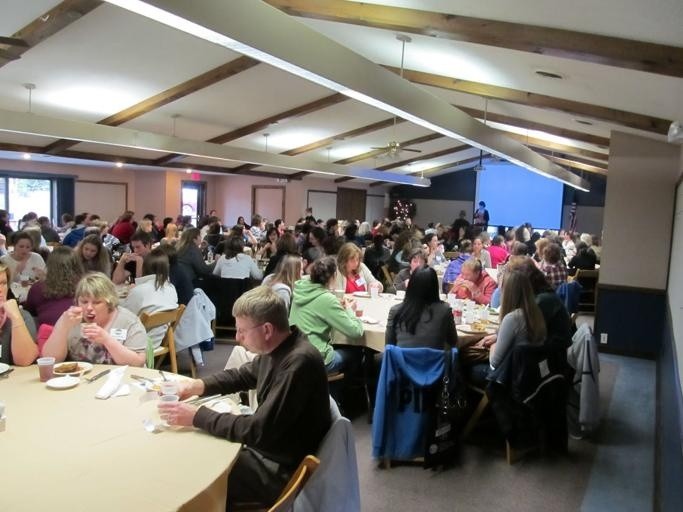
[473,96,487,171]
[667,119,683,146]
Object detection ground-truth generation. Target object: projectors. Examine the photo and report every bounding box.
[474,166,486,171]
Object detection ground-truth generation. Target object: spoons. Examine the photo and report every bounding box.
[79,309,94,318]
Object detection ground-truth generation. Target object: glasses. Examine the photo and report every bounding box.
[234,322,268,333]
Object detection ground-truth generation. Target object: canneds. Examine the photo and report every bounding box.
[453,309,462,325]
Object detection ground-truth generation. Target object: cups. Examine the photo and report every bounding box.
[19,274,29,287]
[156,376,179,428]
[36,357,55,382]
[333,290,498,335]
[81,323,96,338]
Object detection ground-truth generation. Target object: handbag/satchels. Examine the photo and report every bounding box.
[435,344,470,418]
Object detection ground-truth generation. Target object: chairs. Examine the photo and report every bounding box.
[197,240,605,473]
[139,303,187,374]
[227,454,321,511]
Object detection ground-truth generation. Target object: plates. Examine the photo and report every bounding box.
[0,362,9,375]
[51,361,92,375]
[45,377,80,389]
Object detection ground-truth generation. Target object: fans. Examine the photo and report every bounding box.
[370,34,421,159]
